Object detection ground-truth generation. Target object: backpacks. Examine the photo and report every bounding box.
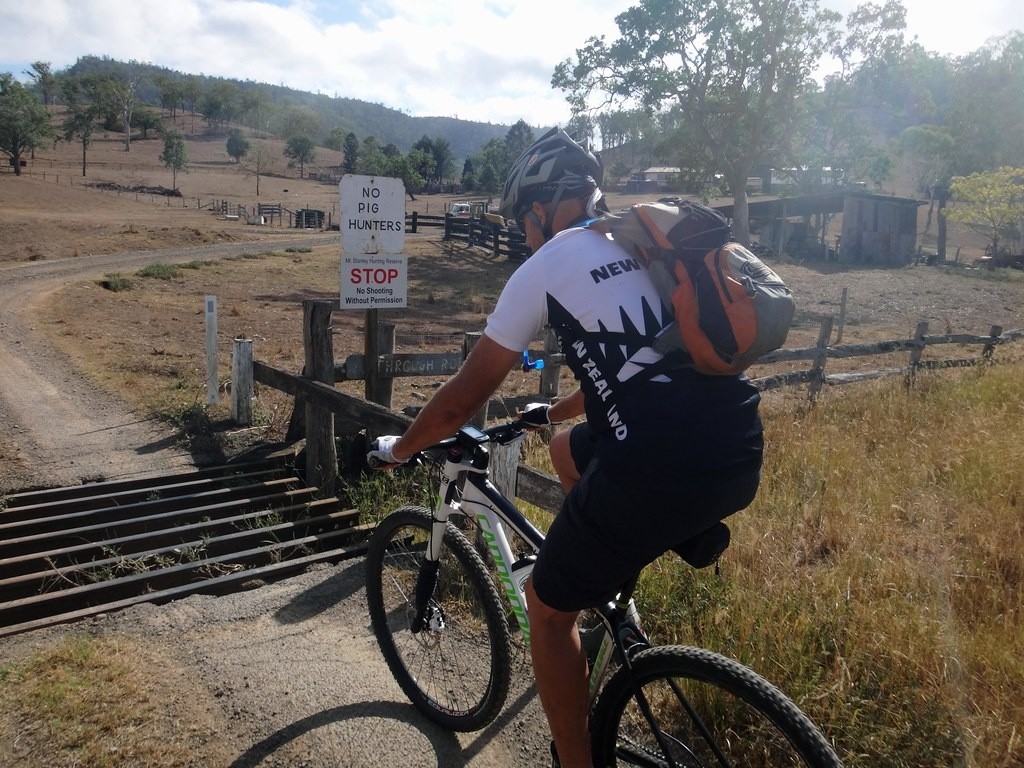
[556,198,795,375]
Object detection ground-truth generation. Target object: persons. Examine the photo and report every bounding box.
[367,124,765,768]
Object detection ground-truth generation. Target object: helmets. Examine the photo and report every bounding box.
[498,126,604,218]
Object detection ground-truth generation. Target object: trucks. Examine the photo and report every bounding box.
[449,201,489,220]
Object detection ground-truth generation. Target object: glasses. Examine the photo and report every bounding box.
[515,207,534,235]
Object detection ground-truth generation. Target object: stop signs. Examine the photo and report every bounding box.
[339,253,408,310]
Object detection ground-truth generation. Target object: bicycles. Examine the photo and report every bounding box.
[362,414,848,768]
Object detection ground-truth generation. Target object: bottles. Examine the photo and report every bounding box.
[512,552,539,612]
